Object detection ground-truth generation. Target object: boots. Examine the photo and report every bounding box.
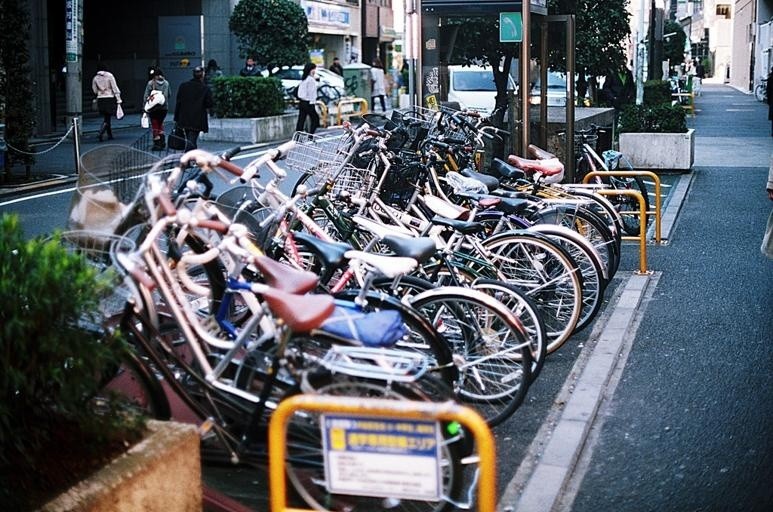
[95,122,109,142]
[158,133,166,148]
[152,137,162,151]
[107,123,115,140]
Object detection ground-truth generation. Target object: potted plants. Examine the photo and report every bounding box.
[0,212,207,512]
[201,75,299,144]
[620,99,697,169]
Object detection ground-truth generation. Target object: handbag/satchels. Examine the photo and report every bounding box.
[168,129,186,150]
[92,99,98,112]
[145,90,165,113]
[116,103,124,120]
[141,113,149,128]
[293,85,302,100]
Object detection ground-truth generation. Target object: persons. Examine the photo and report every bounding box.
[238,57,263,78]
[695,59,704,84]
[92,63,123,142]
[171,65,211,155]
[327,58,342,80]
[291,63,319,143]
[202,59,225,115]
[367,58,389,115]
[141,68,171,152]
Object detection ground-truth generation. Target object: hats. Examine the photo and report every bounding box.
[192,66,207,71]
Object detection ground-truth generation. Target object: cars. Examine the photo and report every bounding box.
[259,65,345,100]
[448,63,566,126]
